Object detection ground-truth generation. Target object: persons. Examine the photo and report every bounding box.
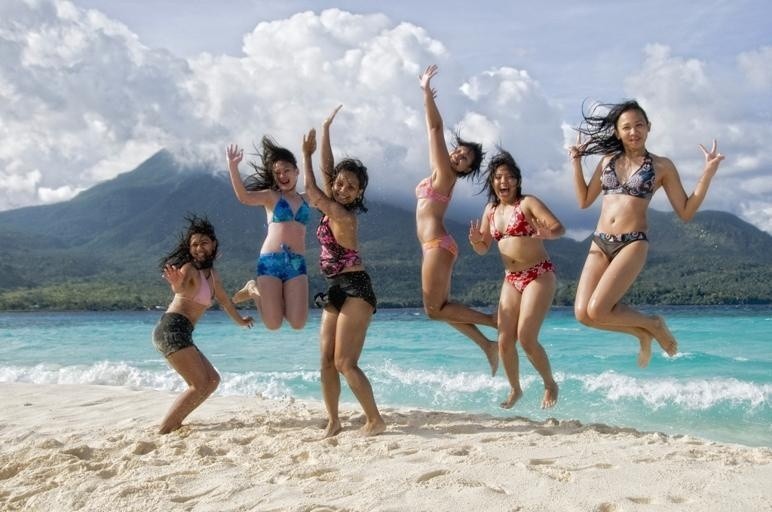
[301,103,386,439]
[414,64,499,376]
[226,142,313,331]
[469,151,566,410]
[151,212,256,438]
[571,98,725,366]
